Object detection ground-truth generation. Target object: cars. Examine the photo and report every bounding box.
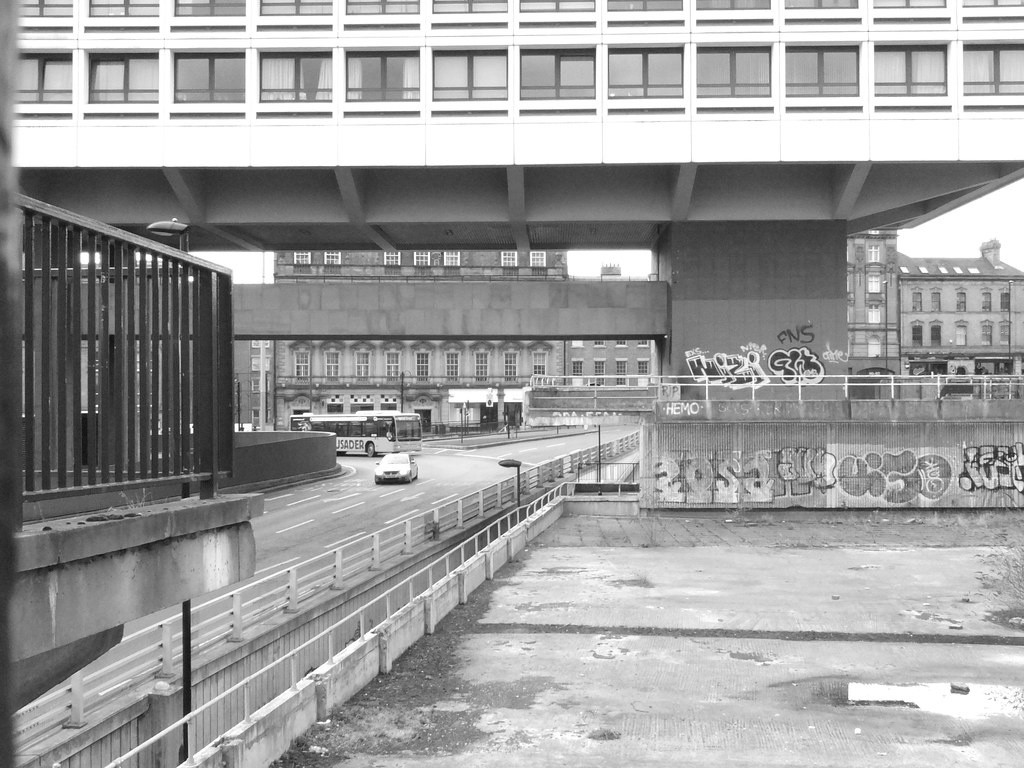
[375,453,418,483]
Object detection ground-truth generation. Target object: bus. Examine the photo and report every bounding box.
[288,410,429,457]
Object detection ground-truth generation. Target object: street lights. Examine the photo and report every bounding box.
[148,222,192,757]
[1008,281,1013,377]
[882,281,888,377]
[234,379,240,431]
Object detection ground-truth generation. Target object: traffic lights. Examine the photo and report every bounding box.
[457,408,461,413]
